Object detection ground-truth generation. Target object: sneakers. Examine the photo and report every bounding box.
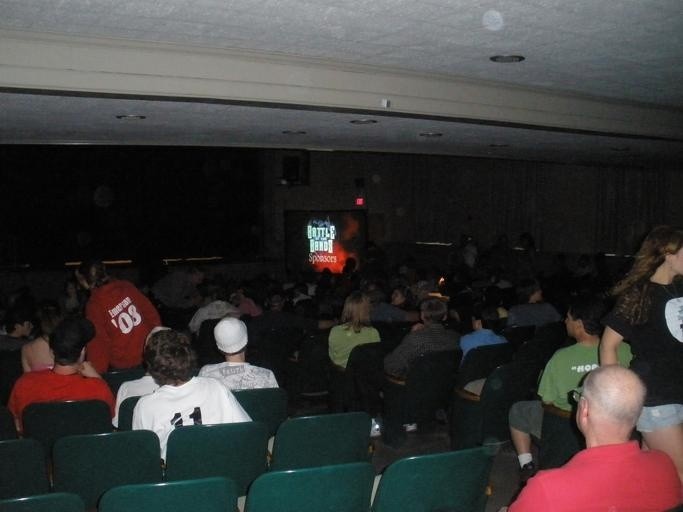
[519,460,537,484]
[369,417,382,436]
[403,421,418,432]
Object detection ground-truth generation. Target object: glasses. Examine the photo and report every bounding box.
[571,388,585,402]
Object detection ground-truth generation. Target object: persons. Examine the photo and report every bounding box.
[509,226,682,511]
[0,256,554,462]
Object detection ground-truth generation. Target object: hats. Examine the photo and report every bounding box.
[48,315,96,360]
[212,316,250,355]
[266,294,286,307]
